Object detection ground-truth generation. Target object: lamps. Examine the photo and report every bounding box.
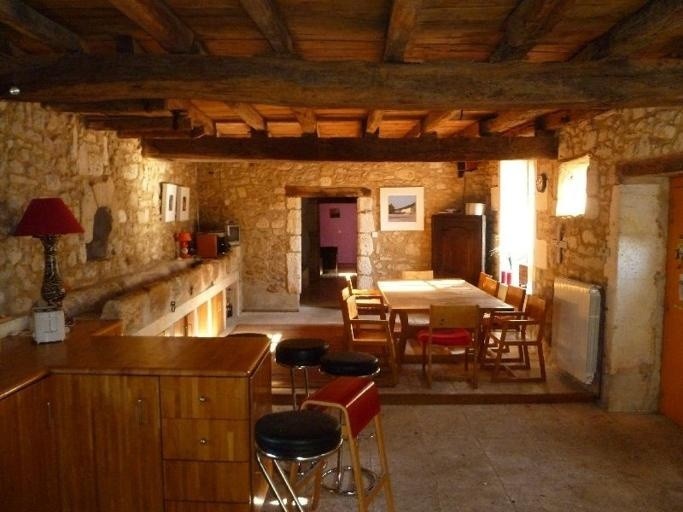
[9,197,87,306]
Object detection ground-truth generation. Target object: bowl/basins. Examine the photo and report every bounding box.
[464,201,487,217]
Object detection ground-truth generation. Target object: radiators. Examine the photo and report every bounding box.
[545,275,602,386]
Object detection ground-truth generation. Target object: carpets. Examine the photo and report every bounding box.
[215,322,400,391]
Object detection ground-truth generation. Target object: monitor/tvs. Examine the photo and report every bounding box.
[212,224,240,246]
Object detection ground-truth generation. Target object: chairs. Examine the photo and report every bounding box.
[337,268,549,390]
[317,245,338,279]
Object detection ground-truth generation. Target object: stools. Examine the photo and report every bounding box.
[316,354,382,496]
[253,409,343,511]
[274,336,332,477]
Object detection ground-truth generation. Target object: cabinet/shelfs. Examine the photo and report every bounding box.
[432,212,486,286]
[0,378,44,511]
[44,375,97,511]
[162,351,270,512]
[98,373,160,510]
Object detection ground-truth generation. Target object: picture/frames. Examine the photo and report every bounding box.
[378,185,424,233]
